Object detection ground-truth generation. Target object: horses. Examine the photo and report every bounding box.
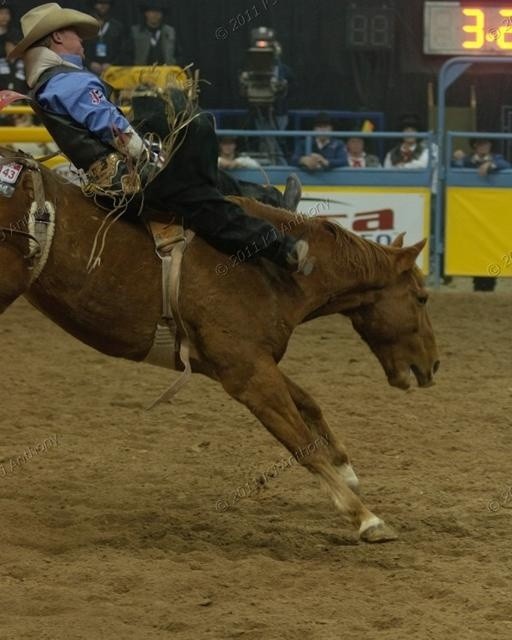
[0,146,442,544]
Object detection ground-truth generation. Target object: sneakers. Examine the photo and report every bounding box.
[279,174,302,213]
[285,240,313,276]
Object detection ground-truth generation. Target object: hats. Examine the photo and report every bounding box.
[398,113,423,129]
[310,112,336,127]
[8,2,99,61]
[136,1,176,9]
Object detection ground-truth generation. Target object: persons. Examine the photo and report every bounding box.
[383,115,428,169]
[218,133,261,169]
[0,1,28,96]
[344,138,383,168]
[452,137,512,291]
[124,2,185,68]
[79,0,128,74]
[287,114,348,172]
[21,27,310,282]
[232,26,295,160]
